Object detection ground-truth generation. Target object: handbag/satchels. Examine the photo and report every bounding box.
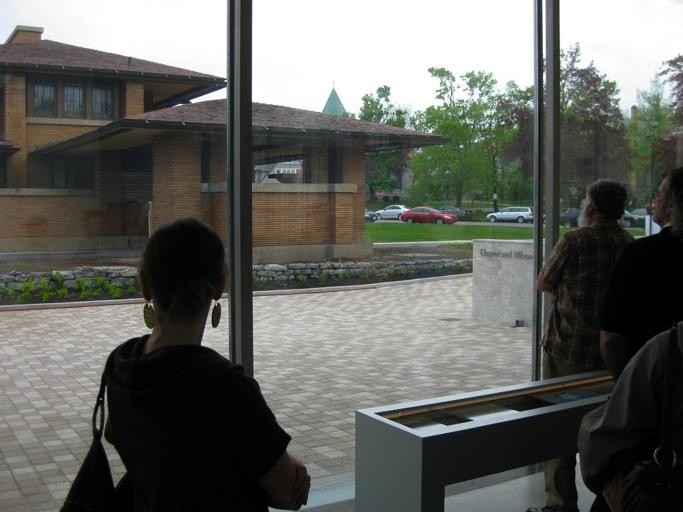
[59,431,114,512]
[603,447,683,512]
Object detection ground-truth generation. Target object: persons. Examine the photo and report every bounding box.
[589,167,682,384]
[528,177,637,509]
[100,214,310,511]
[575,313,683,510]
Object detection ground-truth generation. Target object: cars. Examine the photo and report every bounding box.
[434,205,475,222]
[401,206,458,225]
[365,207,376,222]
[485,204,535,224]
[544,207,655,228]
[374,204,413,221]
